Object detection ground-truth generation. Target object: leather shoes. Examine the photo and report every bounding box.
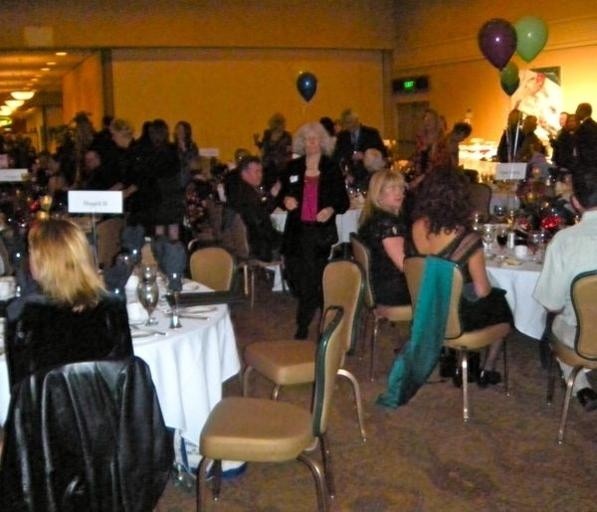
[296,327,308,339]
[576,387,597,411]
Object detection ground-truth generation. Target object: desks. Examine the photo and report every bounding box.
[476,225,555,405]
[482,168,575,215]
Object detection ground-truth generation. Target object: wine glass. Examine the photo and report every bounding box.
[116,245,182,329]
[470,202,542,267]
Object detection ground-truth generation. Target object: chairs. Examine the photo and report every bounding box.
[545,269,595,445]
[402,255,512,424]
[349,231,416,382]
[2,166,367,293]
[243,258,369,442]
[195,304,346,512]
[1,272,230,511]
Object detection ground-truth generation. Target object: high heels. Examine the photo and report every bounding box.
[454,363,480,387]
[477,368,500,387]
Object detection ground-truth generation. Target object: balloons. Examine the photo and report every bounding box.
[296,72,317,102]
[479,18,517,71]
[500,63,519,97]
[513,16,549,63]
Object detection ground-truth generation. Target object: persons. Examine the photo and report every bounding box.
[0,111,210,249]
[359,104,597,411]
[4,215,133,390]
[226,108,389,342]
